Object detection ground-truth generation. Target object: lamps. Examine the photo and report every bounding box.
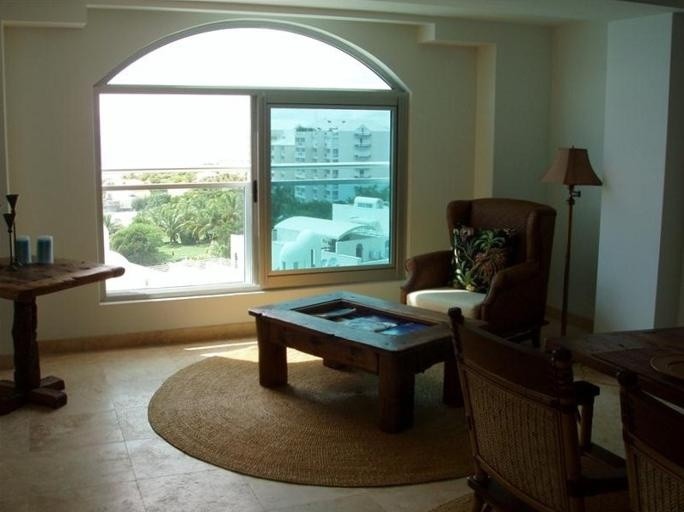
[540,145,603,336]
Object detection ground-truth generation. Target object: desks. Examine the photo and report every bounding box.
[544,327,684,408]
[0,256,125,415]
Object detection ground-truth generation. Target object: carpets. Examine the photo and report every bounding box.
[429,491,485,512]
[148,345,471,490]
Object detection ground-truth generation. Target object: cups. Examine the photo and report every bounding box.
[36,235,55,265]
[14,235,32,265]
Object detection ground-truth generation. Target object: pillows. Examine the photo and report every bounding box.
[449,223,521,293]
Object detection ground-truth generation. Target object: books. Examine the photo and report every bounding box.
[334,314,409,333]
[311,308,356,319]
[374,322,431,337]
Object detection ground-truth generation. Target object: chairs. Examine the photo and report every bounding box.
[400,198,556,348]
[448,307,628,511]
[618,369,684,512]
